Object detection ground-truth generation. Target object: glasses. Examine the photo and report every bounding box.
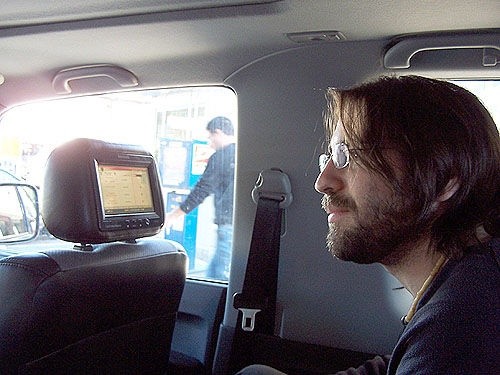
[317,143,414,173]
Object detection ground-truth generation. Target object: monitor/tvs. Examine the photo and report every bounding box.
[88,150,163,231]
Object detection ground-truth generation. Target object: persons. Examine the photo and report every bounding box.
[172,116,236,282]
[231,73,500,375]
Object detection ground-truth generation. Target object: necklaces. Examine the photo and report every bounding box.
[400,251,447,326]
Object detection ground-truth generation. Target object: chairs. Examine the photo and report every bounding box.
[0,138,189,375]
